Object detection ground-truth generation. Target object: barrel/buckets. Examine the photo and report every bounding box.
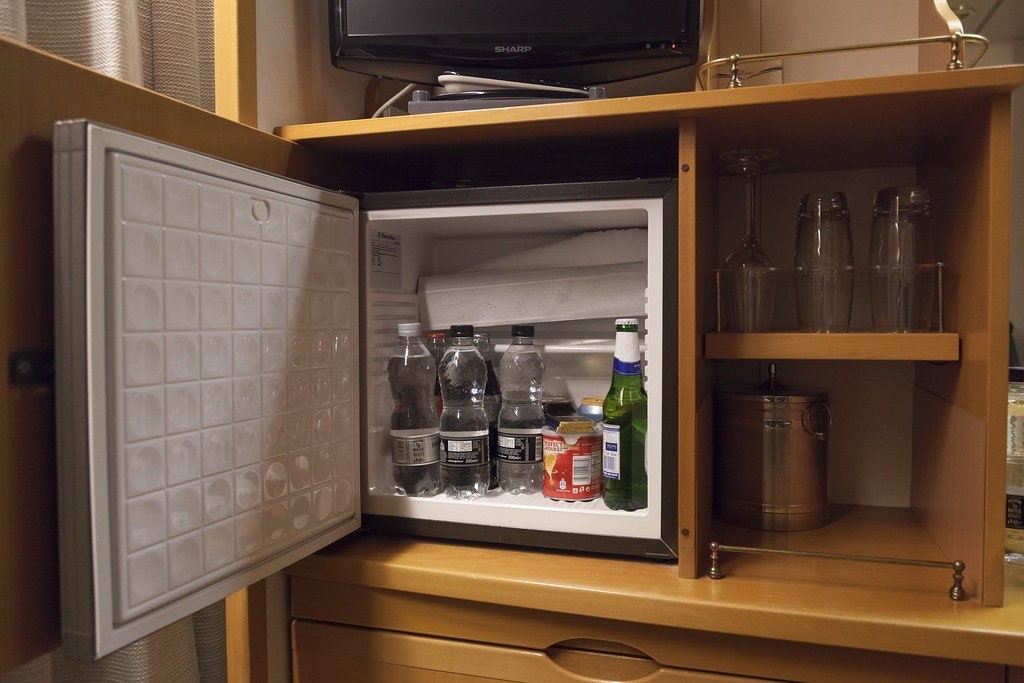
[714,363,832,530]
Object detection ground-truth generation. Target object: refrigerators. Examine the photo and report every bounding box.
[51,118,677,663]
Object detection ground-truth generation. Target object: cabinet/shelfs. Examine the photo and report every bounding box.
[0,0,1024,683]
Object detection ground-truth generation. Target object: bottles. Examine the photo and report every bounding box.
[603,317,647,512]
[387,322,546,499]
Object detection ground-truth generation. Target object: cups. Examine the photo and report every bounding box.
[871,186,937,331]
[795,193,855,332]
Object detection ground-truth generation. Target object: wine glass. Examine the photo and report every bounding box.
[717,145,779,331]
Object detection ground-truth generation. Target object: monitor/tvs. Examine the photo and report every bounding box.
[328,0,702,115]
